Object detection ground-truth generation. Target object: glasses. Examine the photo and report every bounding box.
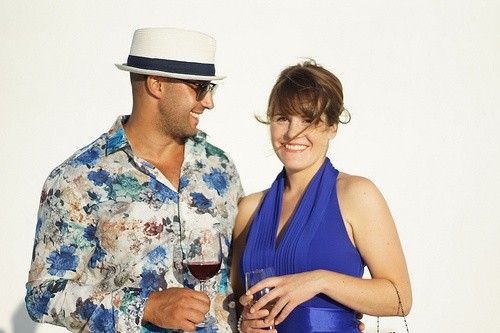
[178,79,220,103]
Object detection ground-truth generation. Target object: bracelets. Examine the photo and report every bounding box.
[237,314,244,333]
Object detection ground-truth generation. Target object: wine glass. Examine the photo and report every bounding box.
[186,229,222,327]
[245,267,279,330]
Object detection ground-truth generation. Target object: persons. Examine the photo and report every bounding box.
[24,25,246,333]
[229,61,413,333]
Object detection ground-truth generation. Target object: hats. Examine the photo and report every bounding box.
[113,27,227,82]
